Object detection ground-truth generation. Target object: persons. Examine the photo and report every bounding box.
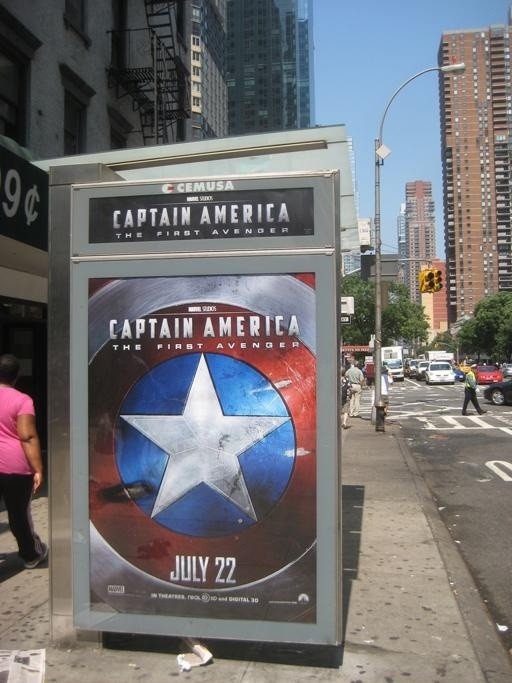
[460,361,487,416]
[0,352,50,574]
[337,347,395,428]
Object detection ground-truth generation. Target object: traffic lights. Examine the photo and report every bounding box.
[434,269,443,291]
[425,272,434,289]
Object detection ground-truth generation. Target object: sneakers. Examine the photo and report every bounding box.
[23,541,49,569]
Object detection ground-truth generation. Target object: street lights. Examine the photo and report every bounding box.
[370,59,467,435]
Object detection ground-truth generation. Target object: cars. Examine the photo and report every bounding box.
[381,344,511,406]
